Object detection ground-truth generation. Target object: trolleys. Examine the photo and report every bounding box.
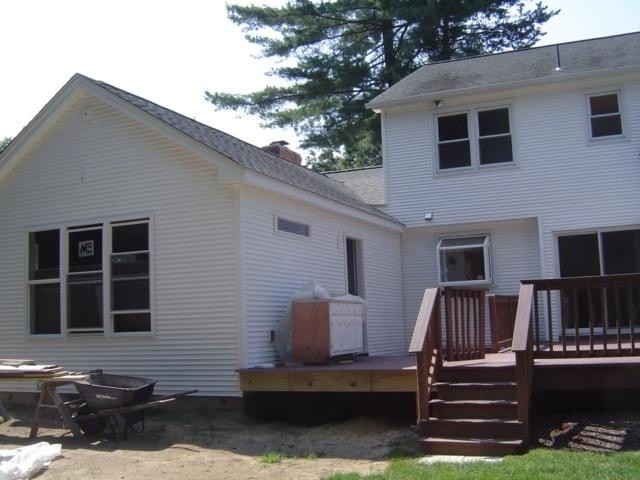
[64,366,201,442]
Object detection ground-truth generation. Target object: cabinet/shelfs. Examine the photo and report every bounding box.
[292,302,364,364]
